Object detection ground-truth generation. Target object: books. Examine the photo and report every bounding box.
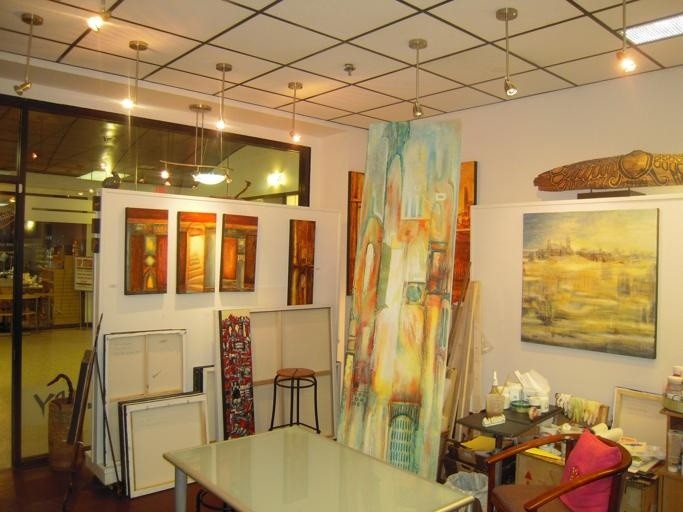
[616,436,660,474]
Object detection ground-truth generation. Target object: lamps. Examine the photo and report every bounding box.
[495,7,519,97]
[159,61,234,186]
[408,37,428,117]
[120,38,150,109]
[85,2,111,33]
[12,12,44,97]
[613,1,637,71]
[286,81,305,143]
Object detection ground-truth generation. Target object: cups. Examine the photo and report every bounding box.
[666,366,683,396]
[667,432,682,472]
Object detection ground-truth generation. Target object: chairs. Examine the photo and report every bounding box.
[0,276,53,334]
[483,431,632,512]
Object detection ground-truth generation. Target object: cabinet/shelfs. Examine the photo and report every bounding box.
[38,254,84,331]
[655,407,683,512]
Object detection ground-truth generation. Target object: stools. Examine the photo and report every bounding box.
[268,366,320,436]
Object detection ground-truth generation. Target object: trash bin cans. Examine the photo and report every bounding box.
[447,471,488,512]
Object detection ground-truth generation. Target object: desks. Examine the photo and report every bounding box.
[162,424,482,512]
[455,398,565,485]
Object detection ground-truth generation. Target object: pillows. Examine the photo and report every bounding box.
[559,424,622,512]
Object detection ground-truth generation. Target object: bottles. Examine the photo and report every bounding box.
[489,371,499,394]
